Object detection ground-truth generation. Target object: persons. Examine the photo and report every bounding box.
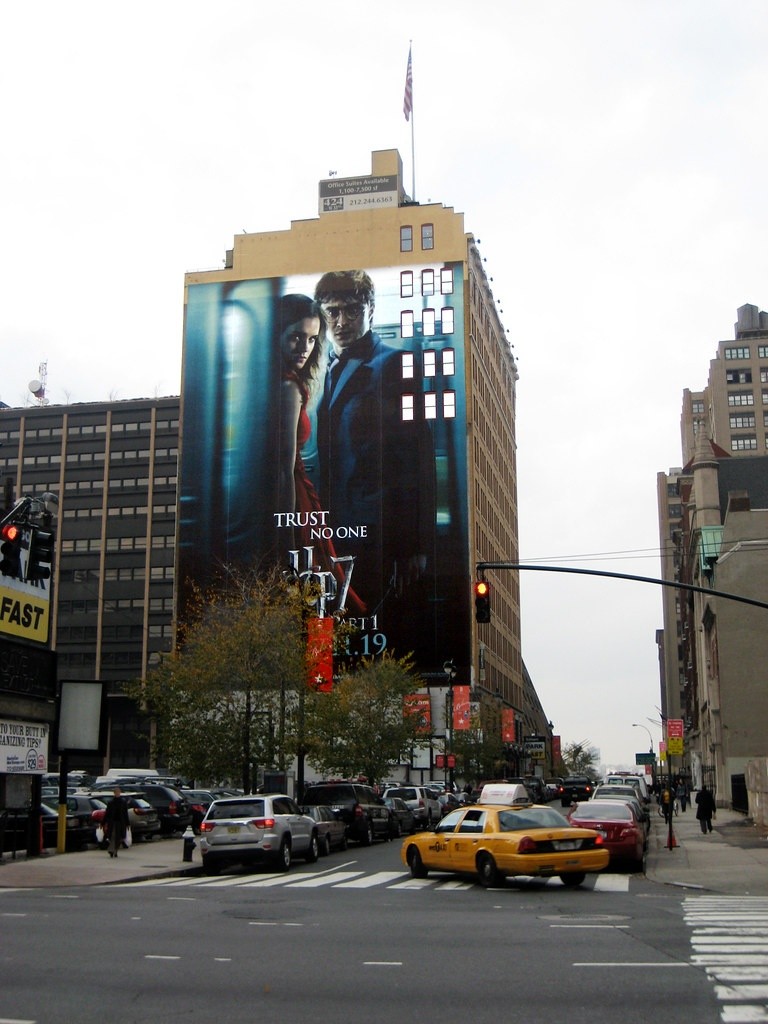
[278,295,368,613]
[314,268,436,611]
[676,779,689,811]
[463,784,472,794]
[695,785,716,834]
[654,779,676,824]
[99,788,130,858]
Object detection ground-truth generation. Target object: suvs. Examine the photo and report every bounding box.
[559,775,592,807]
[194,792,322,879]
[297,780,398,847]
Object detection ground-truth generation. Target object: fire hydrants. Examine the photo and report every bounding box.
[182,824,197,861]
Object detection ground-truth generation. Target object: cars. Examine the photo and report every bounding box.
[297,804,352,857]
[35,766,244,854]
[399,783,611,888]
[375,770,564,840]
[567,771,652,872]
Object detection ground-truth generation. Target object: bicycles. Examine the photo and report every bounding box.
[657,801,679,818]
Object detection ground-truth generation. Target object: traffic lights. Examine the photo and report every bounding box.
[0,523,23,577]
[25,527,53,579]
[475,582,490,623]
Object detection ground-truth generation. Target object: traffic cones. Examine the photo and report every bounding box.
[663,830,679,848]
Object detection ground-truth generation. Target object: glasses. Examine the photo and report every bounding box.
[323,303,369,323]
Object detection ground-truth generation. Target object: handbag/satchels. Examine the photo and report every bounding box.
[96,826,104,842]
[124,827,132,846]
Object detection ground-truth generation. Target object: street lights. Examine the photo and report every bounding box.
[547,720,554,778]
[443,658,458,793]
[632,723,654,755]
[283,561,319,816]
